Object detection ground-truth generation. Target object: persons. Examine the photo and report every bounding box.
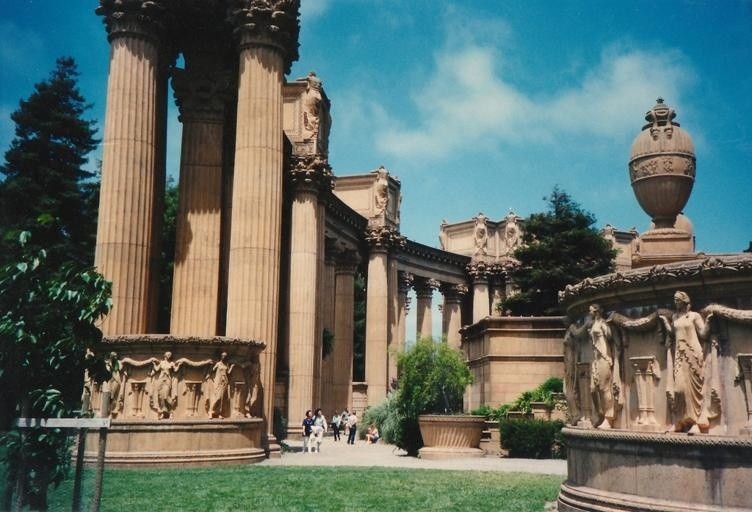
[599,224,618,250]
[329,407,379,445]
[296,72,322,143]
[301,408,329,454]
[205,352,235,419]
[587,303,614,430]
[371,165,389,216]
[472,213,489,255]
[148,351,182,419]
[658,290,714,434]
[104,351,123,419]
[504,212,521,253]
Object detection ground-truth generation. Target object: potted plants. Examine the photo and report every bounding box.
[392,336,489,453]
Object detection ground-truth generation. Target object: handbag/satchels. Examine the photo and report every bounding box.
[302,431,310,437]
